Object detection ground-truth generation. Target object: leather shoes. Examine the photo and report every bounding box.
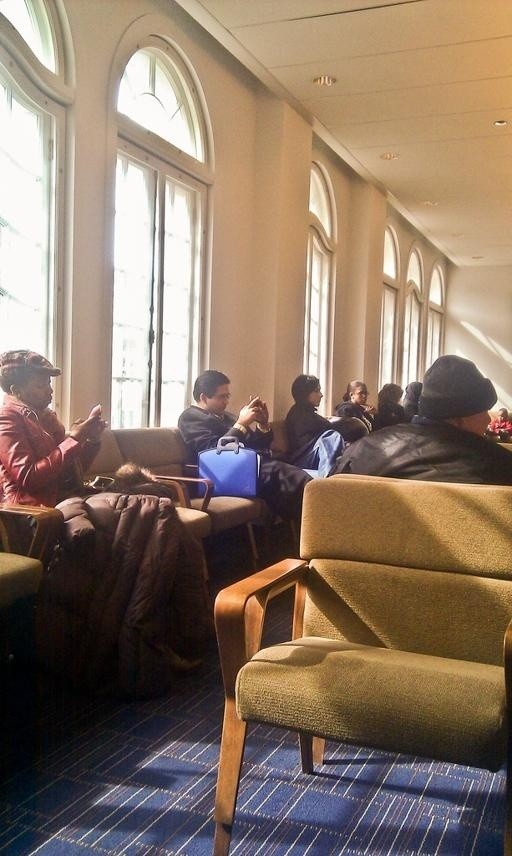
[170,651,202,675]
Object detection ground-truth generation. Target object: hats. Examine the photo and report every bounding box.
[0,349,62,377]
[417,354,498,420]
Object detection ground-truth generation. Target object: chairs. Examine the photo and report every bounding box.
[206,473,511,856]
[0,422,300,660]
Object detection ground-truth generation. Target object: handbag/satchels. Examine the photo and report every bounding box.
[197,435,263,499]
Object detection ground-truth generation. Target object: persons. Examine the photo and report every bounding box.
[285,353,512,486]
[0,347,210,693]
[176,368,312,522]
